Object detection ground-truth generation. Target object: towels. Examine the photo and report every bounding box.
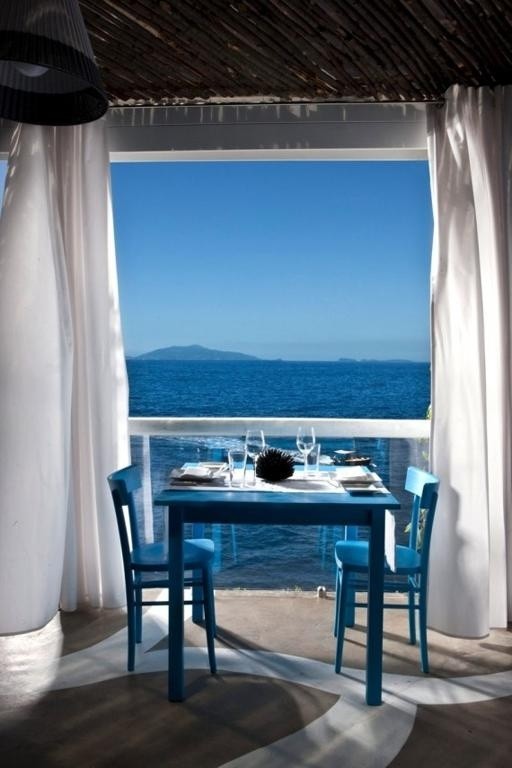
[333,466,369,481]
[180,465,212,479]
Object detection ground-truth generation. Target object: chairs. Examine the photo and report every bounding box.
[174,439,239,574]
[317,435,396,575]
[330,465,443,680]
[107,463,220,679]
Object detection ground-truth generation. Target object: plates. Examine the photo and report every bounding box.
[167,459,386,494]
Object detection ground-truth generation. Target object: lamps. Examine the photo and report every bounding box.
[2,2,109,131]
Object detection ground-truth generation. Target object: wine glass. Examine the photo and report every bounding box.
[225,425,322,489]
